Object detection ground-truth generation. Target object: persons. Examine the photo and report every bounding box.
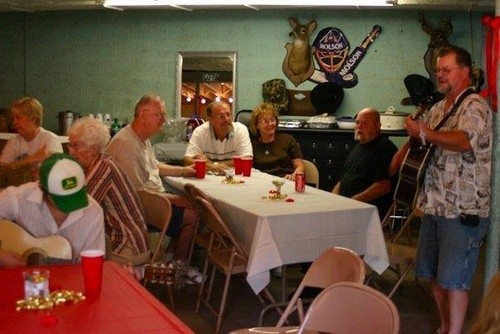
[103,94,210,287]
[66,116,152,268]
[182,96,253,174]
[1,96,66,163]
[248,101,306,279]
[0,108,19,155]
[389,43,493,334]
[288,108,402,304]
[0,152,107,264]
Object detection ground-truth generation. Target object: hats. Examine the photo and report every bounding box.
[39,151,90,210]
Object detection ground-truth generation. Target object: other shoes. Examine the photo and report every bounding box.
[175,262,207,285]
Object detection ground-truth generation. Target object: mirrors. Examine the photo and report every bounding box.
[174,50,238,124]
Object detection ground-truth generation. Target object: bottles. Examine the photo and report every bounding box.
[111,117,121,138]
[96,113,103,123]
[104,113,111,134]
[89,114,94,118]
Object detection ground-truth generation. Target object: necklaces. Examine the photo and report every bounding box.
[260,135,275,156]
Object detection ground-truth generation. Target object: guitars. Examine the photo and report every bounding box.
[0,218,80,267]
[393,94,435,207]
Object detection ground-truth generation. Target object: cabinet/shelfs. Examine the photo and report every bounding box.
[275,127,410,193]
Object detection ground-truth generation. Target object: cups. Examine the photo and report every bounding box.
[233,156,244,175]
[63,110,73,136]
[241,157,253,177]
[195,160,207,179]
[23,269,50,302]
[80,250,106,298]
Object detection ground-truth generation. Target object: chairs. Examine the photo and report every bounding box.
[364,203,424,299]
[181,183,232,312]
[301,160,320,190]
[228,245,367,334]
[194,196,291,334]
[297,282,401,334]
[135,188,176,314]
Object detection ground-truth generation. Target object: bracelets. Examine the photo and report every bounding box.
[180,166,185,178]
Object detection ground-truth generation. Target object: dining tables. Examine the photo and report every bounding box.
[0,259,195,334]
[161,166,392,328]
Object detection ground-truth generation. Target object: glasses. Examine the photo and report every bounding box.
[435,67,464,74]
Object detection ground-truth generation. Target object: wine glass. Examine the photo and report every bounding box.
[272,180,285,198]
